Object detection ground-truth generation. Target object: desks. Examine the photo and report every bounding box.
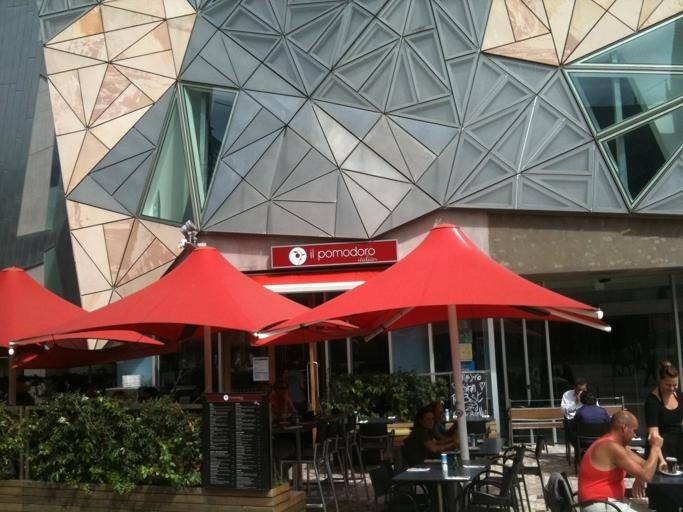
[273,422,317,491]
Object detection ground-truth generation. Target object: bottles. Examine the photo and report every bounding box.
[469,433,477,448]
[445,408,450,422]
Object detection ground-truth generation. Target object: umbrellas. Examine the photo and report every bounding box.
[249,221,614,463]
[0,265,181,404]
[8,244,360,392]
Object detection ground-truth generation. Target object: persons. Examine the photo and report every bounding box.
[644,357,683,470]
[425,400,458,443]
[560,376,599,447]
[266,379,296,423]
[566,388,610,462]
[402,407,458,511]
[577,410,664,511]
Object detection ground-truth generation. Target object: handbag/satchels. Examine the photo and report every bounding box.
[370,460,395,497]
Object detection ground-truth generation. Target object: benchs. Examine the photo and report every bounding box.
[508,396,626,454]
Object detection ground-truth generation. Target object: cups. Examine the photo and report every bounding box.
[440,454,448,464]
[665,457,681,473]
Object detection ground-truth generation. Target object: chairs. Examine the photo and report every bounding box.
[279,414,547,512]
[545,422,646,512]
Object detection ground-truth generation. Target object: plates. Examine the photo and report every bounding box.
[658,470,683,477]
